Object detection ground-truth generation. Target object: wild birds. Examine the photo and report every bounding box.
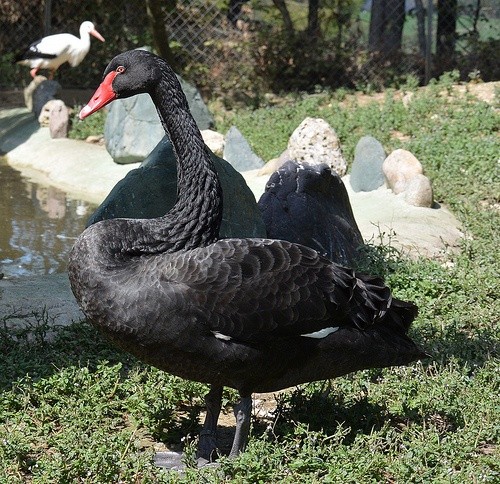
[8,21,106,83]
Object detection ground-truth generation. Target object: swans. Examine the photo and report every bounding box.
[66,47,435,472]
